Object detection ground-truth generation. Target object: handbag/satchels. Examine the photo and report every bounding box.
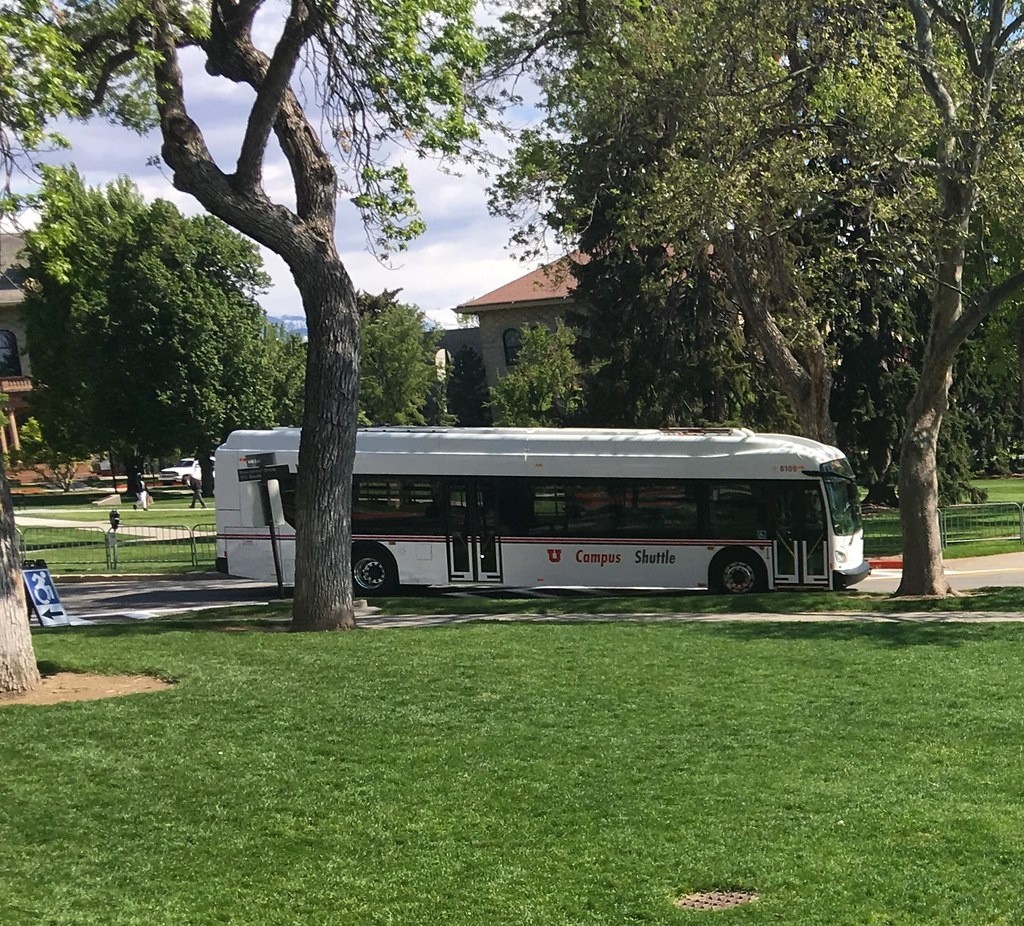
[147,494,155,506]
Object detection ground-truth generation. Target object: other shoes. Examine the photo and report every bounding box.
[189,505,195,508]
[133,504,137,510]
[201,505,207,508]
[144,509,147,512]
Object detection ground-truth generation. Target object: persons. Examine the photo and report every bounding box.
[187,474,207,508]
[133,472,149,511]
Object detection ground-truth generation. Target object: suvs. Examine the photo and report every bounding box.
[157,456,215,486]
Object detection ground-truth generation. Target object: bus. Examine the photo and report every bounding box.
[214,426,874,599]
